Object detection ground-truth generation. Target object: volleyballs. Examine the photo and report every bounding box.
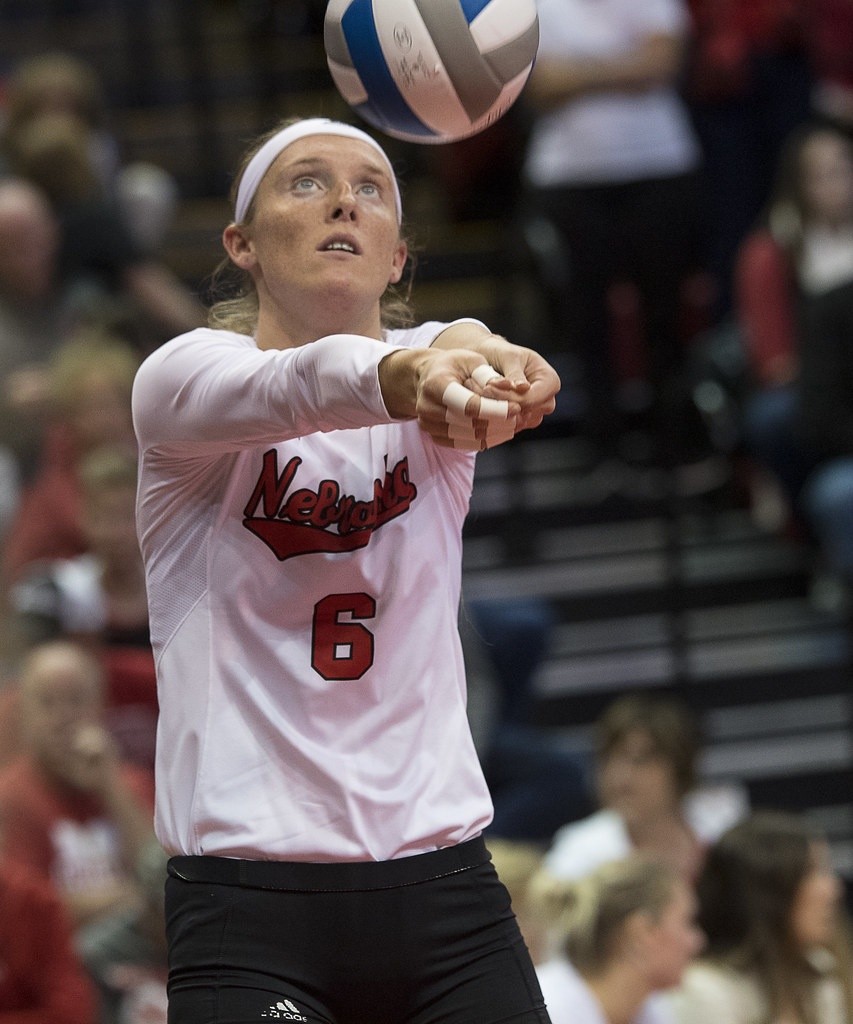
[324,1,540,145]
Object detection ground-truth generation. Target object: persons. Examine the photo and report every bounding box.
[521,692,753,916]
[0,0,853,1024]
[128,119,564,1024]
